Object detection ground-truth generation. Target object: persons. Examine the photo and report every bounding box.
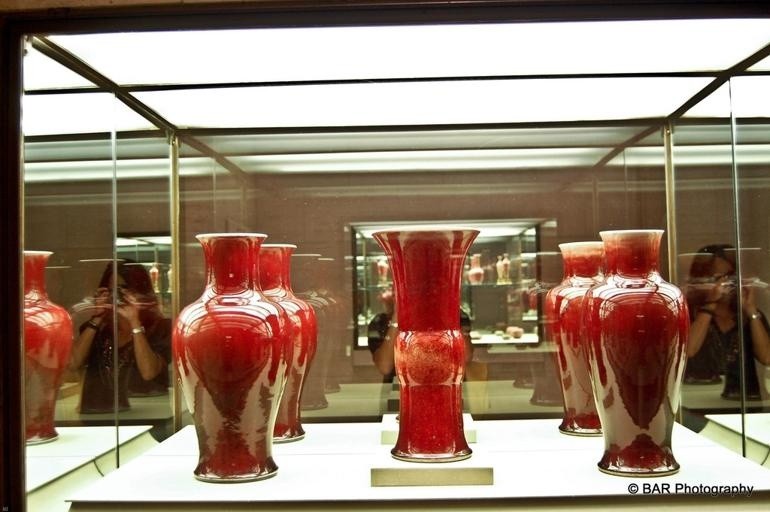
[65,258,174,444]
[678,245,770,434]
[367,309,473,419]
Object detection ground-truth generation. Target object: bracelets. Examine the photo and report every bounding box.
[695,307,716,319]
[747,312,763,322]
[87,319,104,334]
[128,326,148,337]
[387,321,402,330]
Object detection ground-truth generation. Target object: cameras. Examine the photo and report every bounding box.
[713,275,740,297]
[97,285,132,310]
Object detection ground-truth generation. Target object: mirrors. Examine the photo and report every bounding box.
[173,129,671,449]
[21,126,176,426]
[356,225,542,346]
[671,69,770,462]
[670,144,770,415]
[21,33,176,498]
